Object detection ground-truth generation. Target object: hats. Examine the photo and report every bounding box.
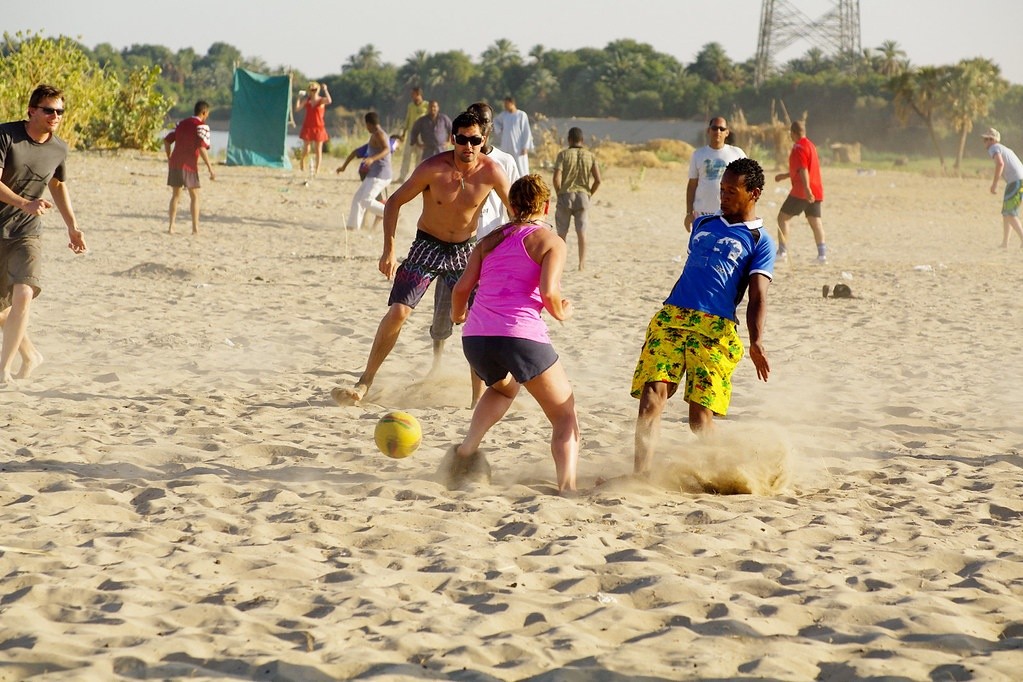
[982,129,1000,141]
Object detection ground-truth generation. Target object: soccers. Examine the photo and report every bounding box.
[374,411,422,459]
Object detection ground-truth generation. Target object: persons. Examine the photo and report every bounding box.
[404,102,520,390]
[448,173,581,495]
[684,112,747,254]
[340,112,515,401]
[774,122,828,265]
[336,112,403,229]
[391,86,430,184]
[630,158,777,472]
[0,82,87,381]
[410,98,452,163]
[980,127,1023,251]
[295,82,332,177]
[494,96,536,179]
[164,100,215,236]
[553,126,601,272]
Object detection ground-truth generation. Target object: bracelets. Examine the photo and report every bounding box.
[687,210,695,215]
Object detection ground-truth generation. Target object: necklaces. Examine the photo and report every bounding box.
[453,153,476,189]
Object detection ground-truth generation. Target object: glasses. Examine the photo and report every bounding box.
[32,106,63,115]
[454,134,482,146]
[711,126,726,131]
